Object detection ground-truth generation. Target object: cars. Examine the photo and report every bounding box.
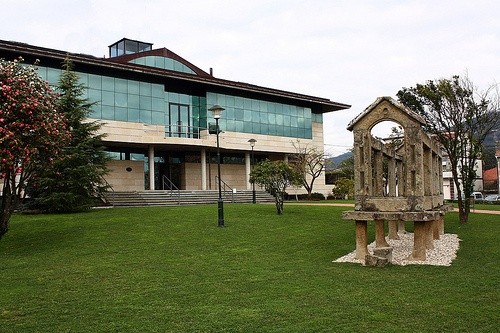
[471,191,499,201]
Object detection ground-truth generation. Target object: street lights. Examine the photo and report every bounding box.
[209,104,226,227]
[248,138,257,205]
